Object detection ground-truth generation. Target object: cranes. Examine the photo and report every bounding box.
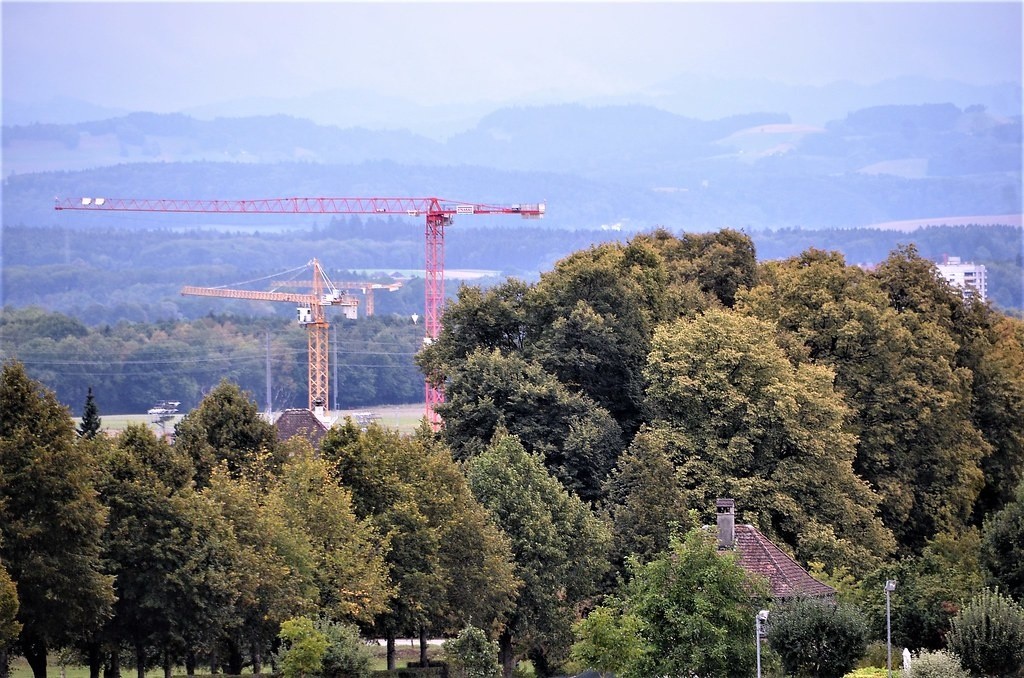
[180,256,403,422]
[52,196,546,432]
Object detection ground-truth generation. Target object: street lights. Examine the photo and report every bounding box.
[884,580,897,678]
[755,610,770,677]
